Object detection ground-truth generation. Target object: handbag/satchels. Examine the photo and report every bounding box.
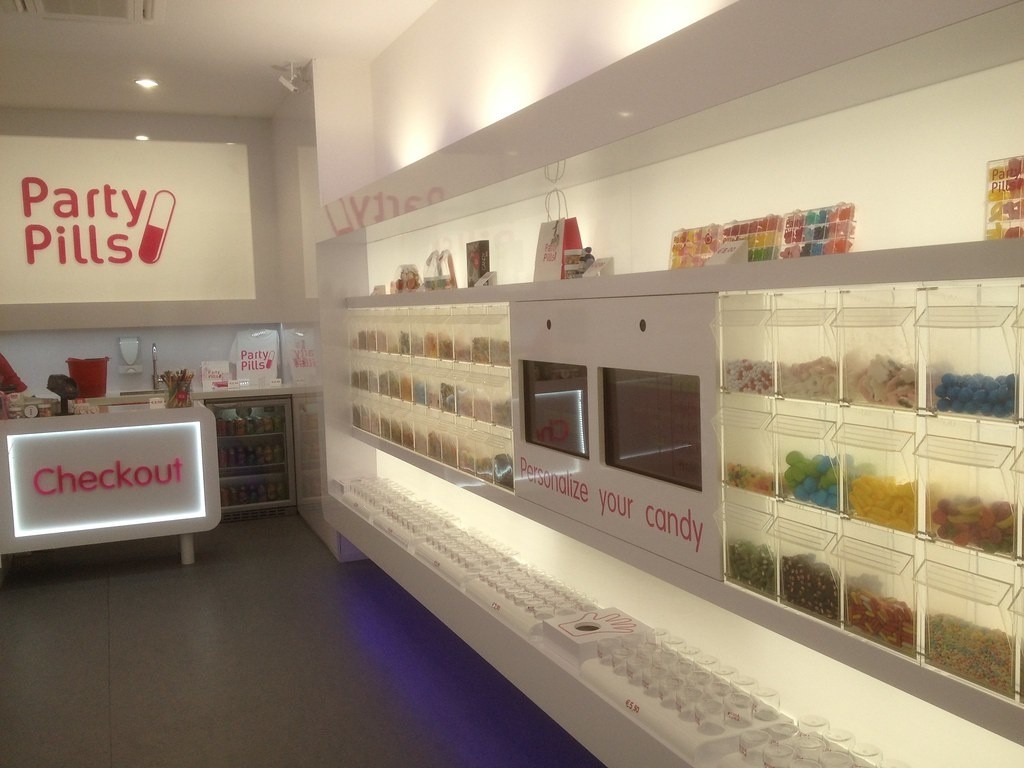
[533,188,583,282]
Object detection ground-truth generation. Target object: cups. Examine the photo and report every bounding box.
[162,380,193,408]
[349,476,599,621]
[598,628,894,768]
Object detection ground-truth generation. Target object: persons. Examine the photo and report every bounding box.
[1,353,28,394]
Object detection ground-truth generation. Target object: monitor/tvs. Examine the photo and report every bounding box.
[523,360,589,460]
[601,367,702,494]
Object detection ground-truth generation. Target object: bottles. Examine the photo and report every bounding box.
[61,386,78,415]
